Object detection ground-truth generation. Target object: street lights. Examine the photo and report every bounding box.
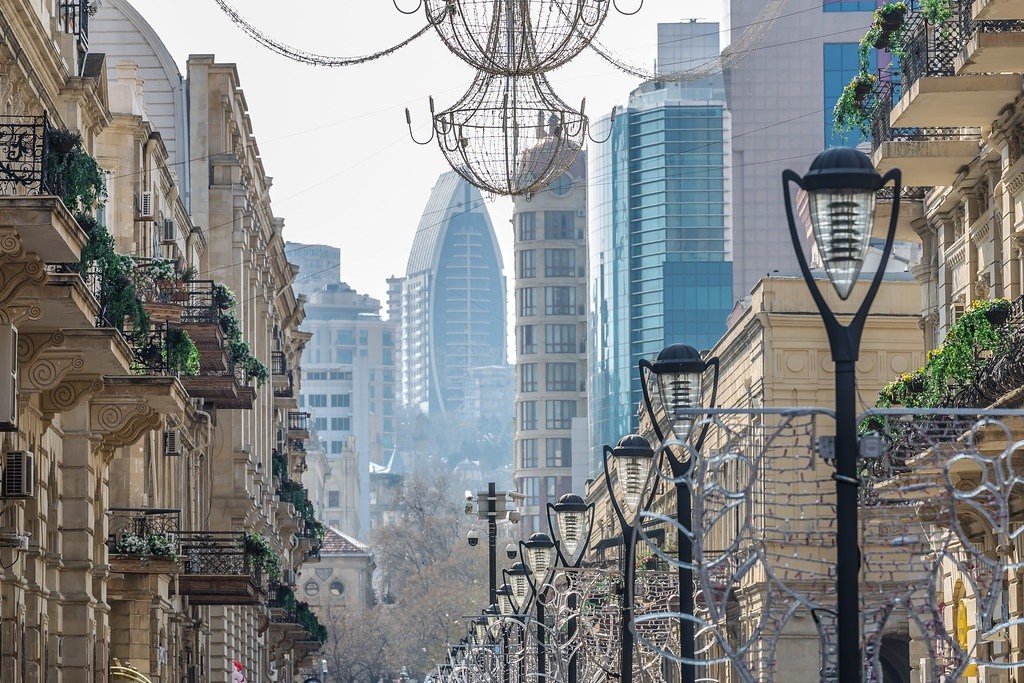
[501,560,538,683]
[637,340,721,683]
[545,492,596,683]
[601,432,663,683]
[781,143,903,683]
[426,582,520,683]
[518,531,561,683]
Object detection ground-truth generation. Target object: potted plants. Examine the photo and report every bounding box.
[832,69,877,136]
[121,533,177,562]
[855,2,906,73]
[43,127,269,384]
[244,532,275,570]
[860,294,1013,437]
[271,444,325,543]
